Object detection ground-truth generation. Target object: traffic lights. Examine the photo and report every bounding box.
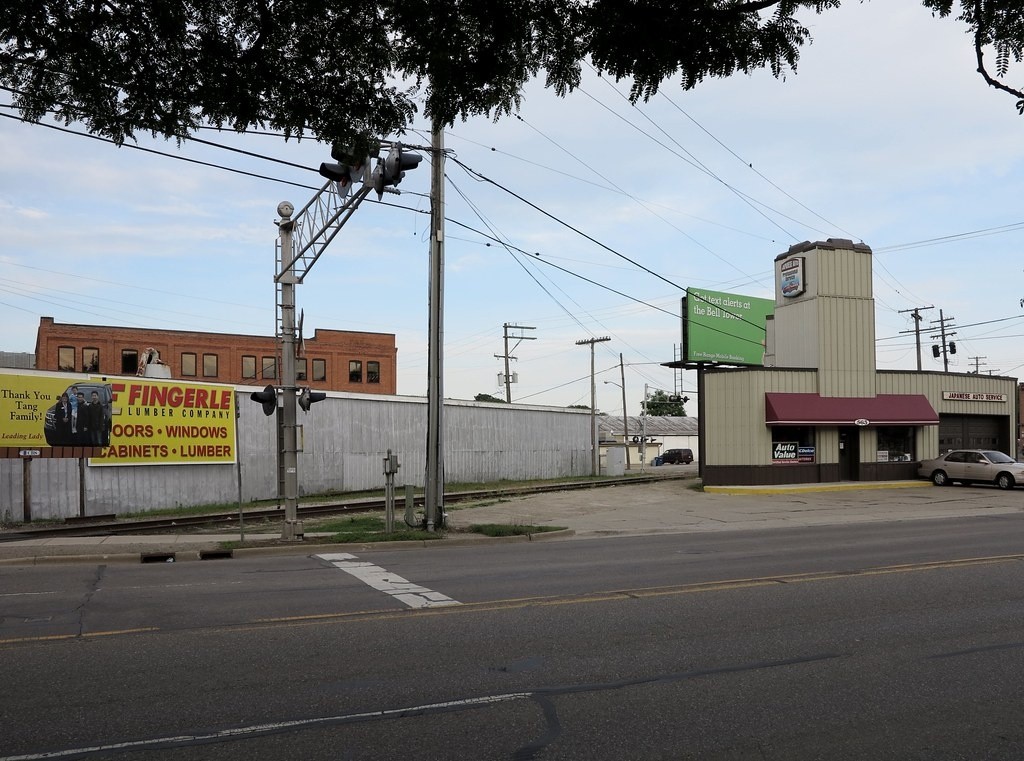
[372,146,422,201]
[319,133,370,198]
[299,386,326,411]
[949,341,956,353]
[249,383,275,416]
[933,344,940,357]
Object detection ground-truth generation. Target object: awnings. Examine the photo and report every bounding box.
[765,392,940,426]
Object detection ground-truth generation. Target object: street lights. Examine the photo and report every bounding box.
[604,352,632,469]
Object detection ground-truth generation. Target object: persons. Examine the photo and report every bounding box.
[56,387,105,446]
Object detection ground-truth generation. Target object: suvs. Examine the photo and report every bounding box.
[44,381,112,447]
[660,448,694,464]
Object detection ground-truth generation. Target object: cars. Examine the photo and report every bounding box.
[919,448,1024,489]
